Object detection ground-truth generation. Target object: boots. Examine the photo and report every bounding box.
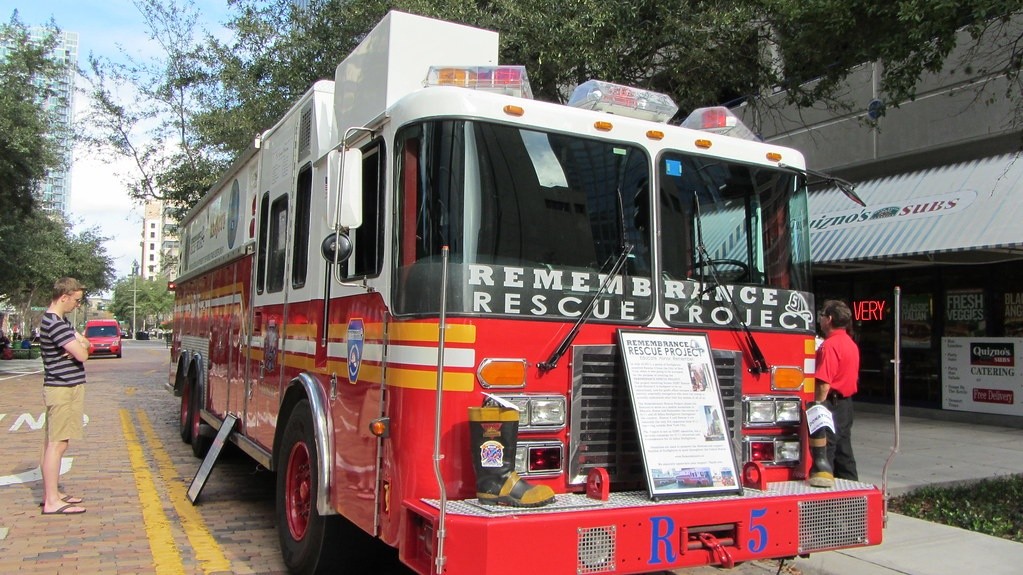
[468,406,557,507]
[806,401,836,486]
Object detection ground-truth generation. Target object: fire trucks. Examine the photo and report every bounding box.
[167,11,900,575]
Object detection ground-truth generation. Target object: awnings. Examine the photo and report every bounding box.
[690,150,1023,275]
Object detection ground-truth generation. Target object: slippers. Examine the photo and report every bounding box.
[41,504,86,514]
[40,495,82,506]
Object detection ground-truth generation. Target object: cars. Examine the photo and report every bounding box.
[83,319,125,358]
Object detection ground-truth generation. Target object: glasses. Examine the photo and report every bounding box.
[818,310,828,317]
[63,293,81,303]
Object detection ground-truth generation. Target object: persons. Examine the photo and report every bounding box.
[815,299,860,481]
[0,320,40,360]
[39,277,91,514]
